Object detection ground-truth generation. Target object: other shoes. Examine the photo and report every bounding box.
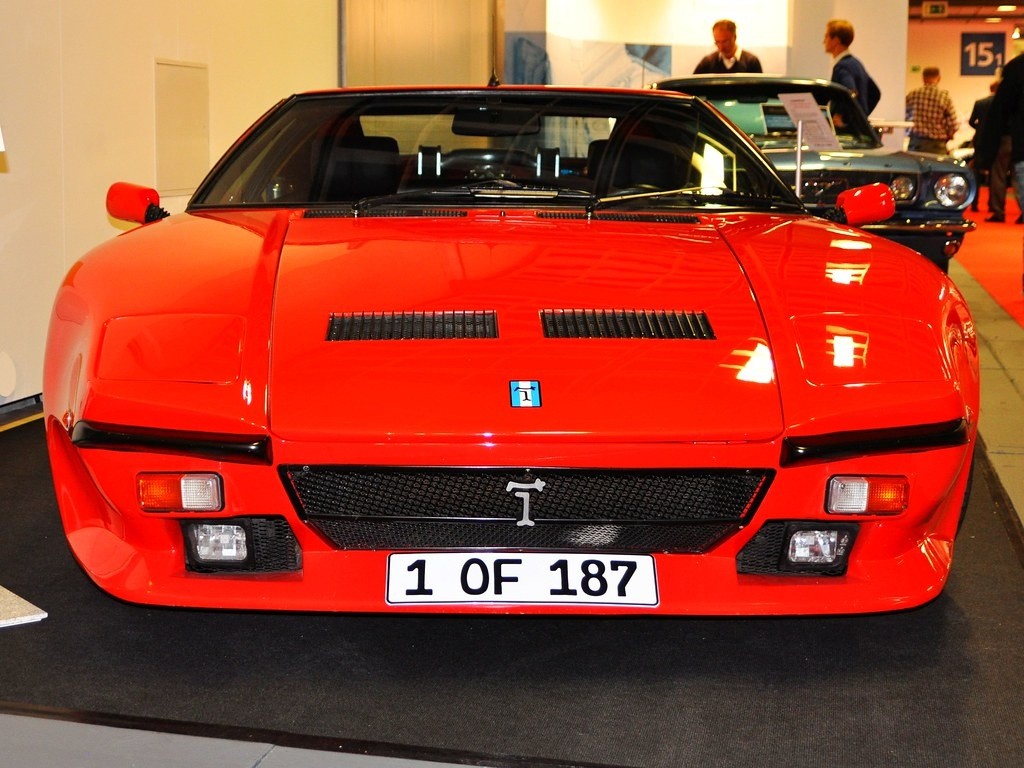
[988,208,994,212]
[1015,212,1024,224]
[985,214,1004,222]
[971,206,977,212]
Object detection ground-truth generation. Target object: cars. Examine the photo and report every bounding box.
[42,64,981,616]
[646,73,976,275]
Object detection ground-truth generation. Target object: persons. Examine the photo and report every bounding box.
[968,52,1024,223]
[906,68,958,155]
[823,19,881,127]
[693,20,762,74]
